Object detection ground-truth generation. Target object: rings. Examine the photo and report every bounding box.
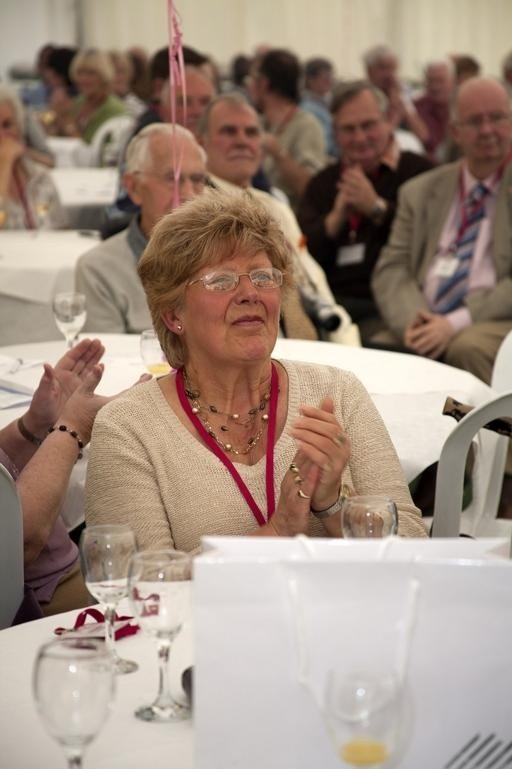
[321,461,334,471]
[289,463,310,499]
[333,433,347,445]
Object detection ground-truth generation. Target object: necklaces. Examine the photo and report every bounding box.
[184,373,272,454]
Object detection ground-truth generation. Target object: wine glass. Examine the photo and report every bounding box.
[125,546,198,726]
[33,636,116,768]
[51,291,87,352]
[76,523,143,676]
[29,181,58,241]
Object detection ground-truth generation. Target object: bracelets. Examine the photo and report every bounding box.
[17,417,44,446]
[310,484,351,518]
[47,424,84,464]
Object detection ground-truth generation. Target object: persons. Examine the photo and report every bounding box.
[371,79,511,474]
[1,43,512,343]
[84,192,430,583]
[1,338,153,618]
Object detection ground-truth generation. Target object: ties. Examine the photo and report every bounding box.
[429,184,488,314]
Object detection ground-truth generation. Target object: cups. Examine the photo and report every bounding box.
[340,494,399,539]
[308,668,412,768]
[140,329,177,377]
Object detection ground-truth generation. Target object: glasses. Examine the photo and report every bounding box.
[189,266,290,296]
